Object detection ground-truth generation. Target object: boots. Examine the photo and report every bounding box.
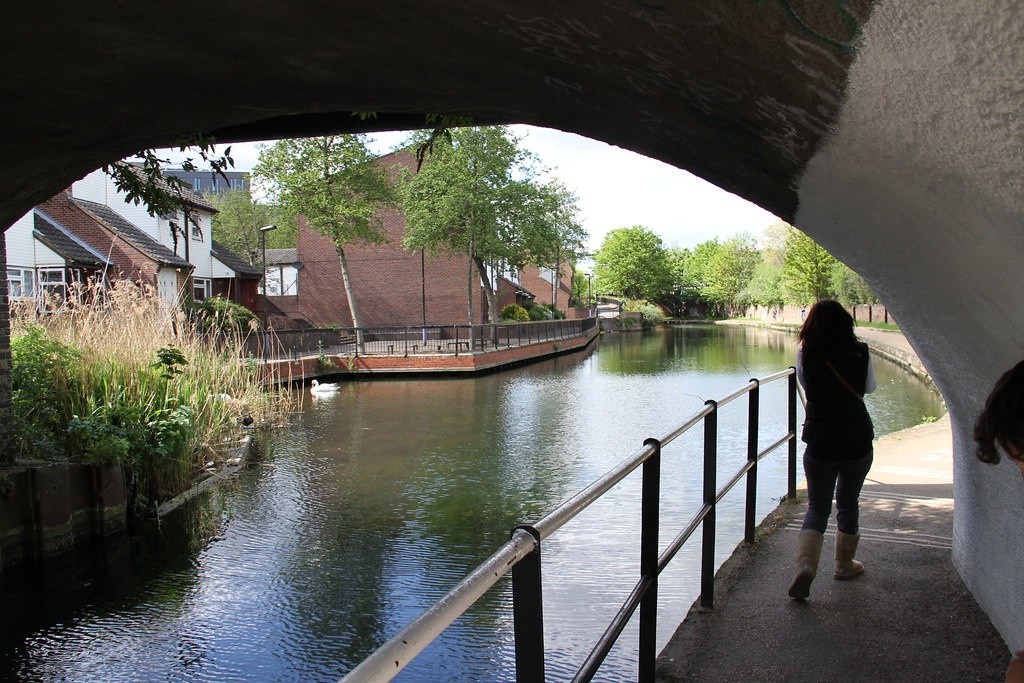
[788,529,824,599]
[834,529,865,580]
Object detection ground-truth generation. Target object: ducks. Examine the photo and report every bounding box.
[310,379,340,393]
[209,394,232,400]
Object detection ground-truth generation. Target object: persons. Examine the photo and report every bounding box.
[788,300,876,598]
[973,359,1024,683]
[801,305,805,322]
[772,308,777,319]
